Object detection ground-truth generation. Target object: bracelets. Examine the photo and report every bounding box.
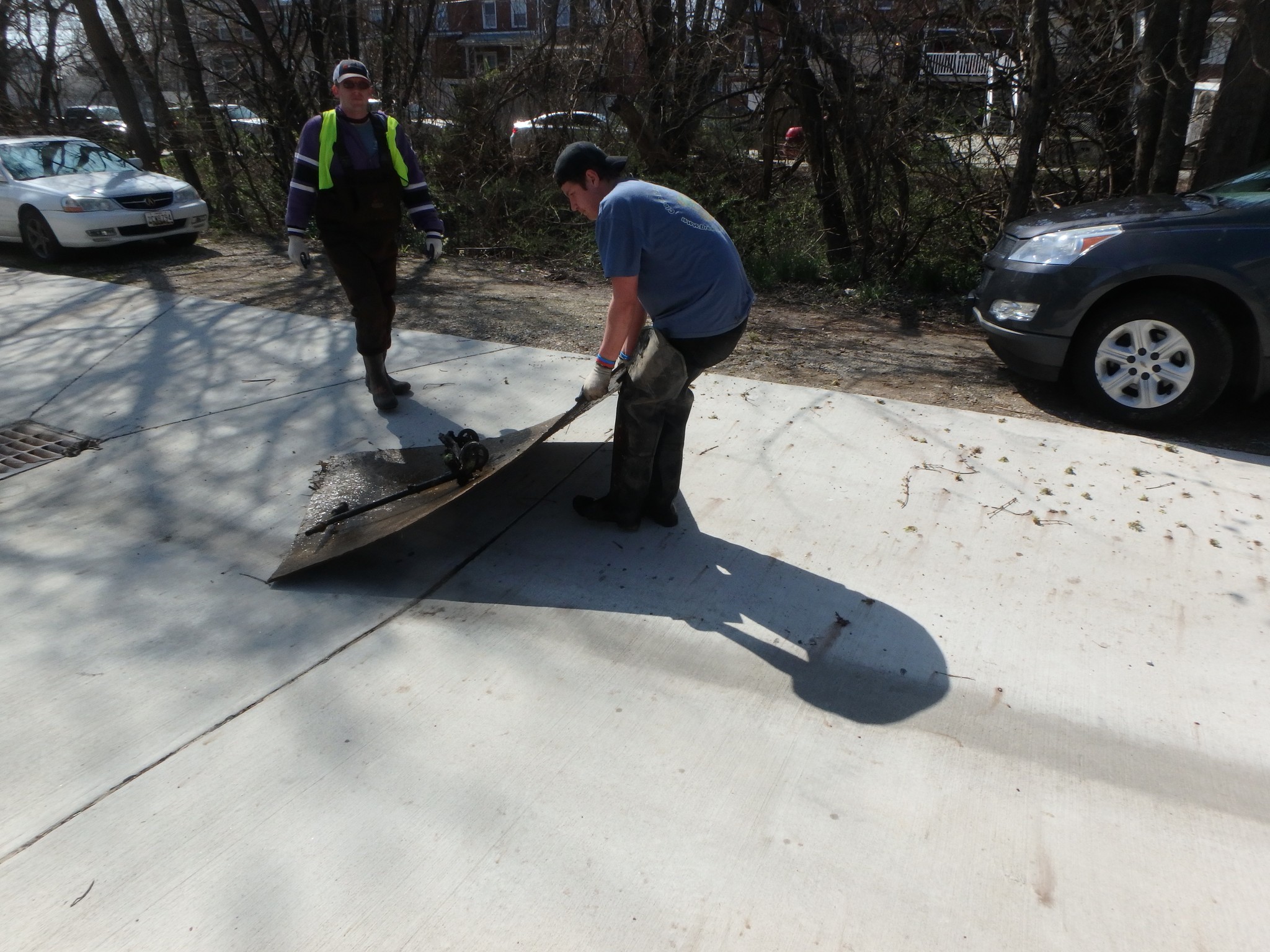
[619,351,627,358]
[597,355,615,368]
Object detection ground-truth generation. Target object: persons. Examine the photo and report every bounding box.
[553,141,755,532]
[284,59,444,410]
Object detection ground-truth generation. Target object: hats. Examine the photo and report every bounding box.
[332,60,371,83]
[552,141,627,181]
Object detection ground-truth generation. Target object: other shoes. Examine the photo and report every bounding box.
[647,504,678,528]
[573,493,642,530]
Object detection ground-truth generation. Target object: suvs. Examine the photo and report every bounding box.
[63,105,161,140]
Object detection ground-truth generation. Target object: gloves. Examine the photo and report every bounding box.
[575,354,616,402]
[613,349,632,377]
[289,235,310,272]
[425,230,444,262]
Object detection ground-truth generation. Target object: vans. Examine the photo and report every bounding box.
[1130,78,1224,169]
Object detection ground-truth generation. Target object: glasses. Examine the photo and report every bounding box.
[342,81,372,89]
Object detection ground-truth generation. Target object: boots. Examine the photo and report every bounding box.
[362,350,399,410]
[362,351,411,395]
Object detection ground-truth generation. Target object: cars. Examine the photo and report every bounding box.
[167,103,274,144]
[0,136,208,263]
[511,111,632,154]
[332,99,463,137]
[966,166,1270,432]
[782,113,878,162]
